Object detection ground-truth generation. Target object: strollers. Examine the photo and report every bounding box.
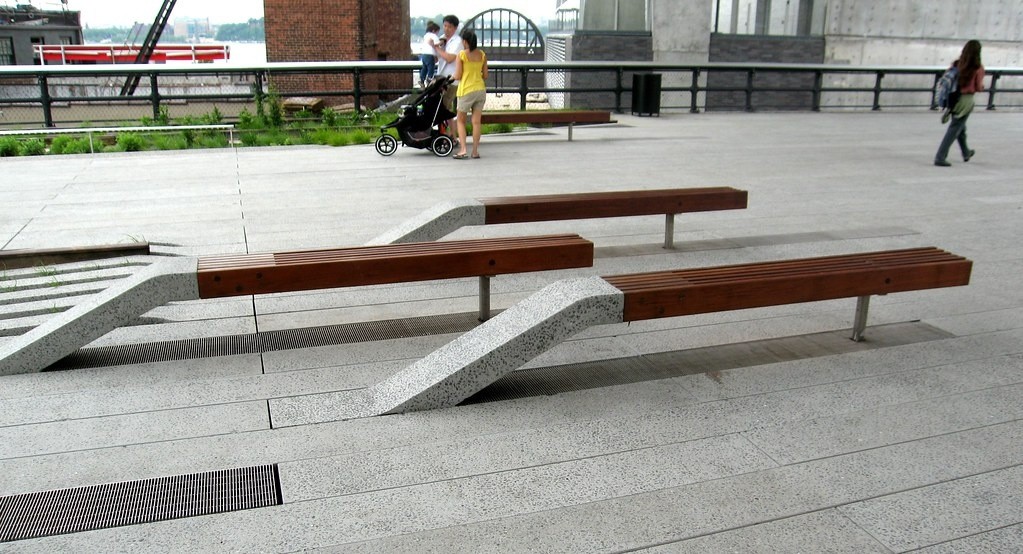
[375,75,458,157]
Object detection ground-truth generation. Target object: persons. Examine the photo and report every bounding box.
[934,39,985,167]
[428,15,464,147]
[418,21,441,87]
[449,30,488,159]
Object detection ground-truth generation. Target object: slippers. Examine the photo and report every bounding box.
[453,153,468,159]
[471,153,480,158]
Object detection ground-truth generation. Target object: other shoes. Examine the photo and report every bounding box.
[418,80,425,89]
[964,149,975,162]
[453,140,458,147]
[935,161,951,166]
[425,78,432,84]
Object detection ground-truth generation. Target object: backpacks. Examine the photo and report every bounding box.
[934,60,980,108]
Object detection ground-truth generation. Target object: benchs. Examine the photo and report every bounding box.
[375,247,973,415]
[481,110,611,140]
[0,233,593,376]
[368,185,748,247]
